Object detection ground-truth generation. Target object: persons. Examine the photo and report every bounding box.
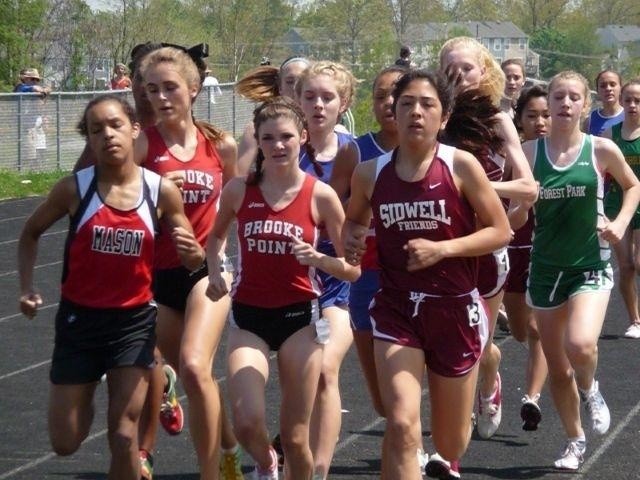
[93,62,108,90]
[110,62,132,89]
[73,42,188,480]
[32,100,50,168]
[14,93,207,480]
[128,46,246,480]
[13,70,28,92]
[12,67,51,167]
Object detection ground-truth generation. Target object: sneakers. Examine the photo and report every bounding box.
[159,364,183,437]
[474,370,504,441]
[624,322,640,339]
[253,444,278,480]
[496,308,511,334]
[416,447,429,477]
[424,453,461,480]
[219,443,245,480]
[136,448,154,480]
[520,393,543,432]
[553,429,587,471]
[577,378,612,435]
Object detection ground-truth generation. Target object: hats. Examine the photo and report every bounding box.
[21,68,45,80]
[401,44,416,54]
[113,62,129,75]
